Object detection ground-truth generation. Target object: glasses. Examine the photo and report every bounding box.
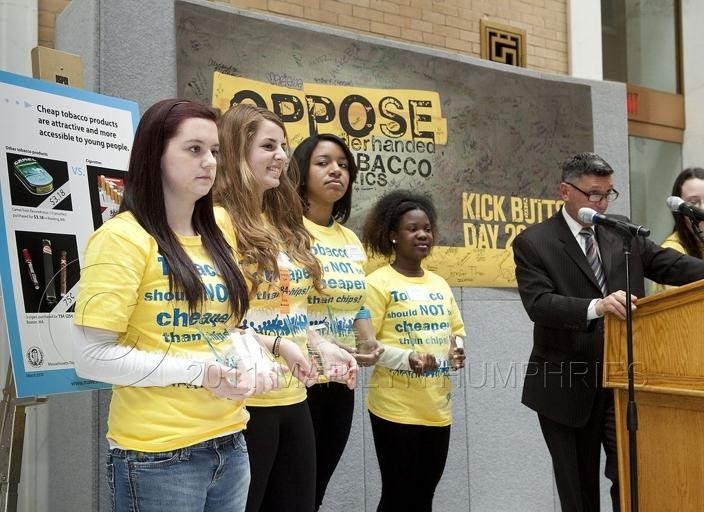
[567,182,619,202]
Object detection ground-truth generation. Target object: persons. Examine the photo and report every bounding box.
[508,151,703,512]
[650,166,704,294]
[70,96,290,510]
[359,188,467,512]
[277,132,387,511]
[210,102,359,512]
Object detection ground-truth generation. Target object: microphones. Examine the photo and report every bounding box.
[577,207,650,238]
[666,196,704,221]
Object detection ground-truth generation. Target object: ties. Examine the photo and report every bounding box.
[578,228,608,295]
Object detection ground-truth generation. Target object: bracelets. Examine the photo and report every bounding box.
[270,334,279,354]
[273,336,282,357]
